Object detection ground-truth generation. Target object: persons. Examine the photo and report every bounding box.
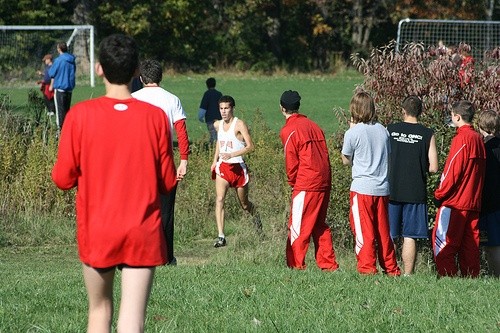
[341,91,402,277]
[130,59,190,265]
[49,41,76,141]
[280,90,339,272]
[478,110,500,277]
[432,100,487,278]
[51,34,179,333]
[439,41,474,88]
[198,78,223,145]
[386,95,439,275]
[36,55,56,117]
[210,96,263,247]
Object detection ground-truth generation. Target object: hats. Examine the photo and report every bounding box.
[42,53,53,60]
[280,89,302,109]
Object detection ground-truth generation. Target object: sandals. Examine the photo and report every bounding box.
[252,217,264,232]
[212,235,227,247]
